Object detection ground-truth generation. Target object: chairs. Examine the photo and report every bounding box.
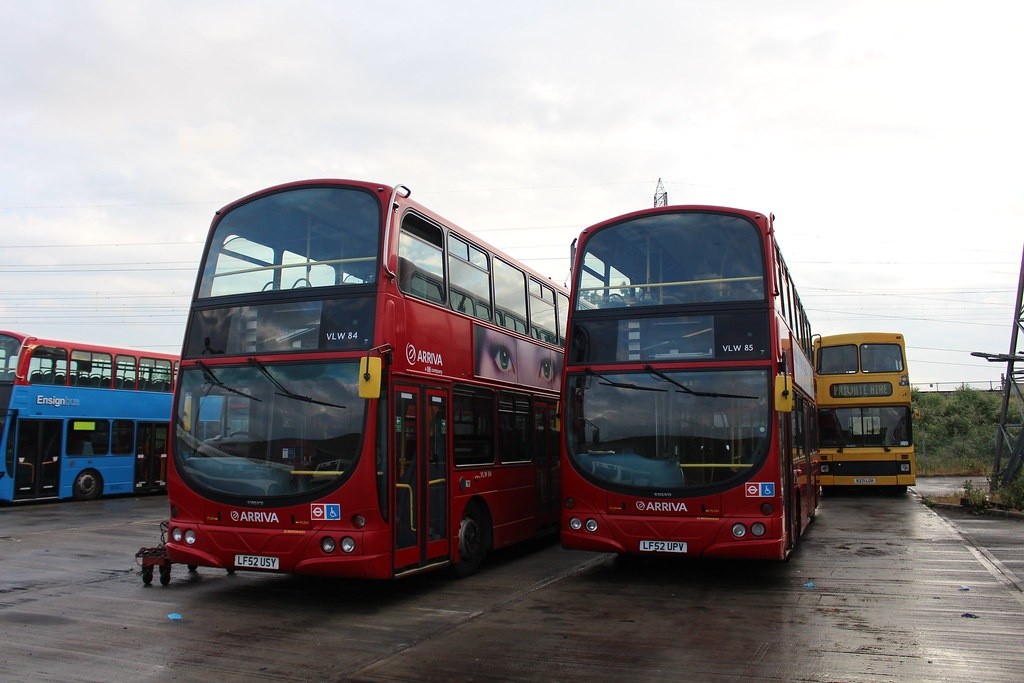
[262,278,312,291]
[0,368,170,392]
[599,292,657,307]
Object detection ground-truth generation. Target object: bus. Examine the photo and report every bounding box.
[811,331,918,498]
[0,329,252,503]
[558,177,823,568]
[133,177,598,603]
